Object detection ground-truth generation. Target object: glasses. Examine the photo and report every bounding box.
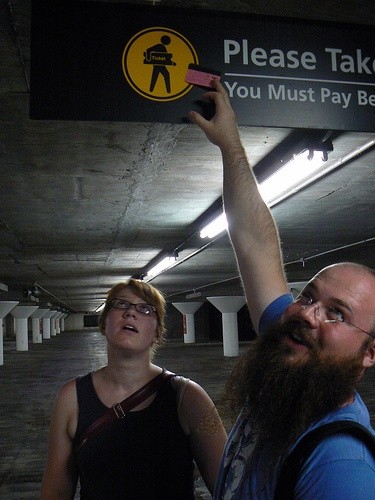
[111,298,156,314]
[291,288,375,339]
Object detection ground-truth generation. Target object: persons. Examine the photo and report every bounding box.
[45,278,229,500]
[189,77,375,500]
